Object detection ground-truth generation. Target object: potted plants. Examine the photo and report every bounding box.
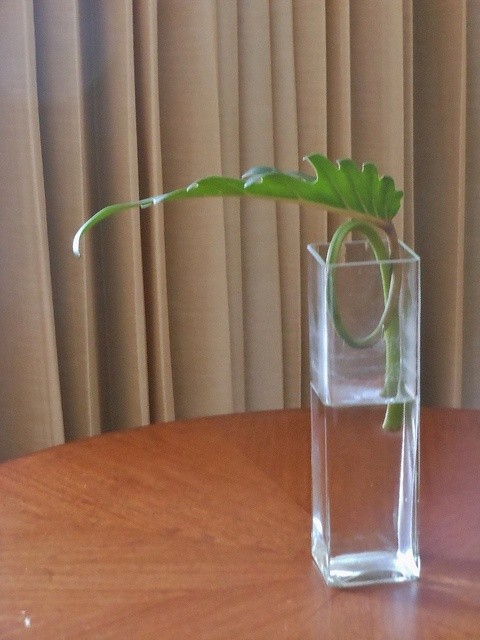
[72,152,424,586]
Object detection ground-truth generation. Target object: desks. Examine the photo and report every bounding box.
[0,408,480,639]
